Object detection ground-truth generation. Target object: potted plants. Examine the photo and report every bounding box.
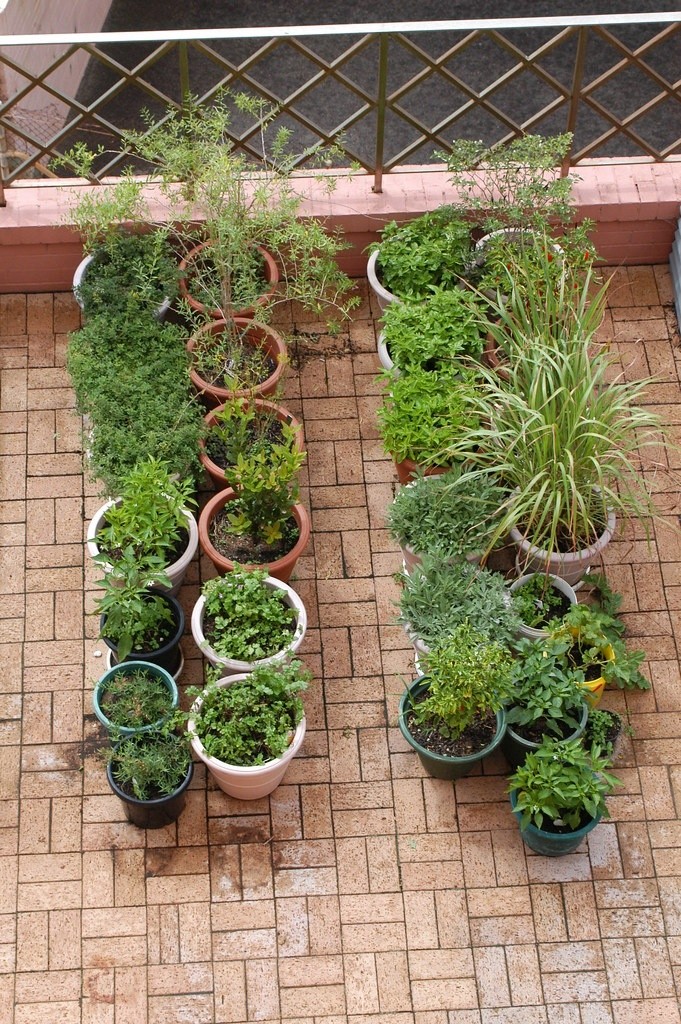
[46,85,364,599]
[440,323,681,589]
[473,227,569,305]
[542,602,650,709]
[190,561,307,677]
[444,223,637,381]
[376,280,490,388]
[359,204,470,313]
[390,548,526,677]
[382,465,510,582]
[503,734,623,857]
[371,361,491,486]
[506,636,592,759]
[94,708,196,830]
[182,658,314,801]
[507,572,578,641]
[397,617,522,780]
[88,544,185,681]
[87,661,178,748]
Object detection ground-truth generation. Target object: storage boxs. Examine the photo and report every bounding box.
[585,707,622,757]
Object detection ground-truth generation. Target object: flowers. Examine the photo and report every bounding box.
[430,131,607,298]
